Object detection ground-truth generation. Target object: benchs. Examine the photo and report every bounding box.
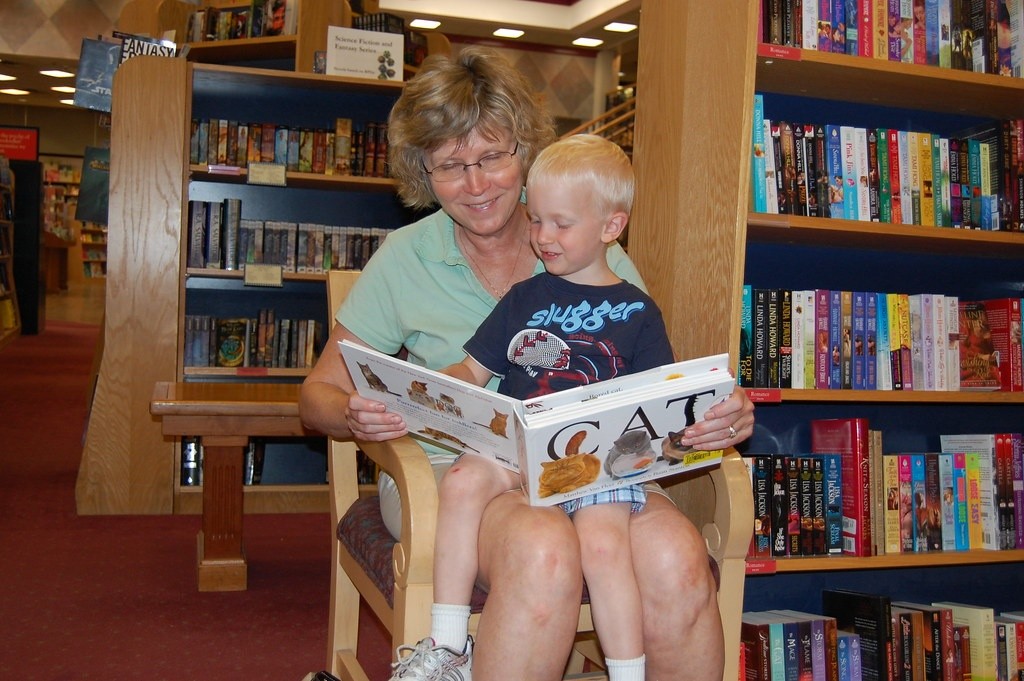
[149,380,378,592]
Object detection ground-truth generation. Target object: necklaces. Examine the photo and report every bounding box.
[458,216,529,300]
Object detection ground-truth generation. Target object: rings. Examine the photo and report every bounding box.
[728,425,737,438]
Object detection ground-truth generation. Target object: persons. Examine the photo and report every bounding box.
[900,15,913,57]
[892,22,901,38]
[913,1,925,30]
[826,24,833,44]
[946,649,953,663]
[860,176,867,187]
[868,333,877,355]
[959,302,1003,387]
[995,17,1012,48]
[808,190,818,205]
[847,179,855,186]
[818,21,826,42]
[833,29,840,44]
[888,487,898,511]
[837,23,845,45]
[943,487,954,506]
[388,134,676,681]
[869,168,877,183]
[834,177,843,203]
[888,14,896,37]
[843,329,851,359]
[854,333,863,356]
[816,172,824,183]
[832,345,840,368]
[965,31,972,57]
[297,44,756,681]
[953,27,961,53]
[830,184,841,202]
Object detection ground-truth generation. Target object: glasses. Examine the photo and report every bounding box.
[421,141,518,182]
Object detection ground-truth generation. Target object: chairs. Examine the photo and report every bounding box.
[301,269,754,681]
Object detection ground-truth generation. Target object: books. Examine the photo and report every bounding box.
[336,337,736,508]
[184,308,323,369]
[188,0,299,41]
[326,446,376,486]
[190,116,390,179]
[0,160,106,330]
[187,198,396,275]
[180,434,205,486]
[243,439,267,487]
[351,12,429,67]
[739,0,1024,681]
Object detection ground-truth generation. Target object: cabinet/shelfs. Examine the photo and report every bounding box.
[75,56,410,515]
[626,0,1024,681]
[118,0,352,74]
[0,157,23,351]
[67,201,108,298]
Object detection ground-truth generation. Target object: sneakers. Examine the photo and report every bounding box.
[387,634,474,681]
